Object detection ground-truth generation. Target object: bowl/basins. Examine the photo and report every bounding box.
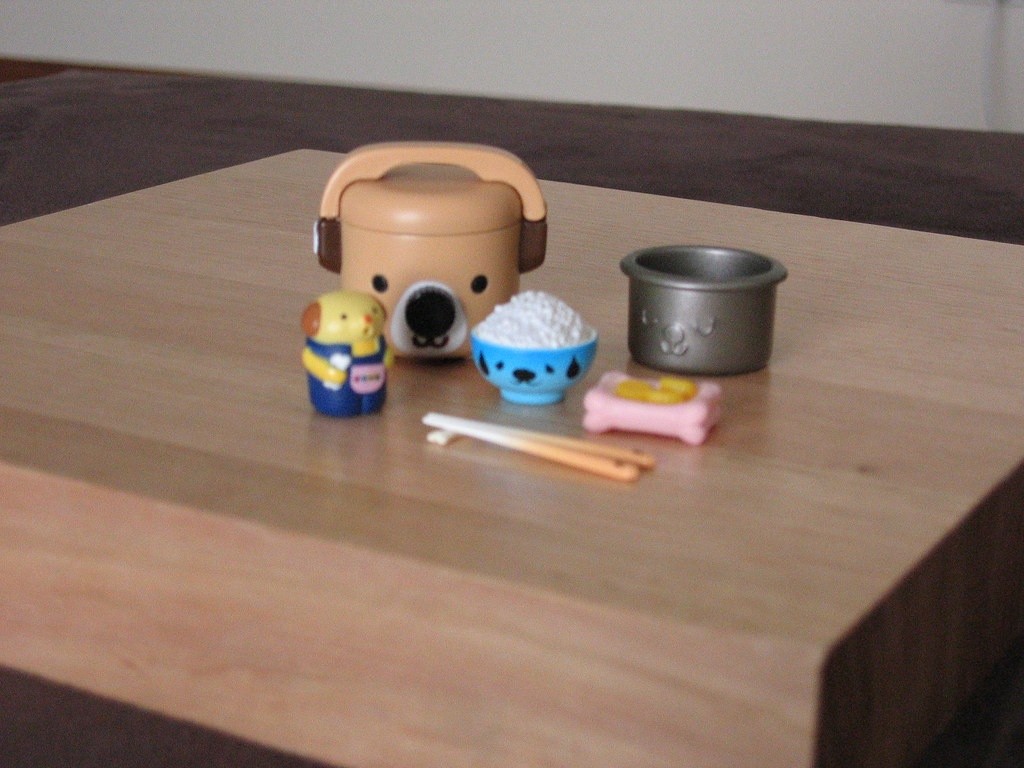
[470,327,598,406]
[621,246,788,373]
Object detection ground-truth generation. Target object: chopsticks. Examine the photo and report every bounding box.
[424,411,655,482]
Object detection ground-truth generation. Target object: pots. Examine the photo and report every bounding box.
[319,141,546,358]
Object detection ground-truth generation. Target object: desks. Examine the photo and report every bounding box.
[0,148,1024,768]
